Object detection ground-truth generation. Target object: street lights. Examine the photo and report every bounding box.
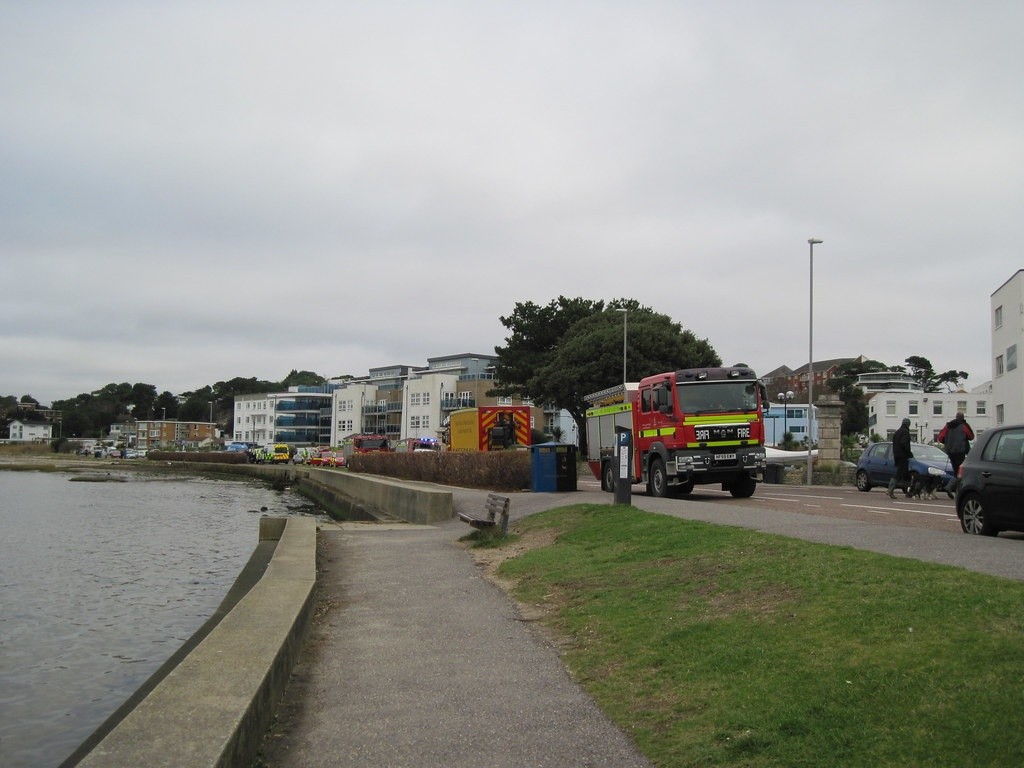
[272,393,277,444]
[806,236,824,486]
[616,309,627,384]
[777,390,795,451]
[134,418,137,449]
[126,421,129,448]
[208,401,213,451]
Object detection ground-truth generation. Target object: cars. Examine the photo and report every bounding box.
[80,441,146,459]
[228,441,346,468]
[954,424,1024,537]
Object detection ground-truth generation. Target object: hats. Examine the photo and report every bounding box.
[902,418,910,425]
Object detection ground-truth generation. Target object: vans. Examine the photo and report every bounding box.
[855,441,966,497]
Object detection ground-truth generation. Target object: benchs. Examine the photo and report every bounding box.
[457,493,511,536]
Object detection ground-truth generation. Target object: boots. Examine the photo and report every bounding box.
[886,478,898,499]
[944,476,957,499]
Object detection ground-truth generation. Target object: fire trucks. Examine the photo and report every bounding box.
[582,363,770,499]
[342,431,391,469]
[395,437,441,453]
[440,405,531,452]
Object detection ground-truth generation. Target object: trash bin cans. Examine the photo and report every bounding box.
[530,442,580,493]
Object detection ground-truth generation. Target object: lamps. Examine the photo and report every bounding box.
[923,397,928,402]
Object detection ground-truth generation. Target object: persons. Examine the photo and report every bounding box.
[886,418,913,499]
[938,412,975,499]
[85,447,127,459]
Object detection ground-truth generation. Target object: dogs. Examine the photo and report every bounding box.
[922,467,958,501]
[901,468,924,500]
